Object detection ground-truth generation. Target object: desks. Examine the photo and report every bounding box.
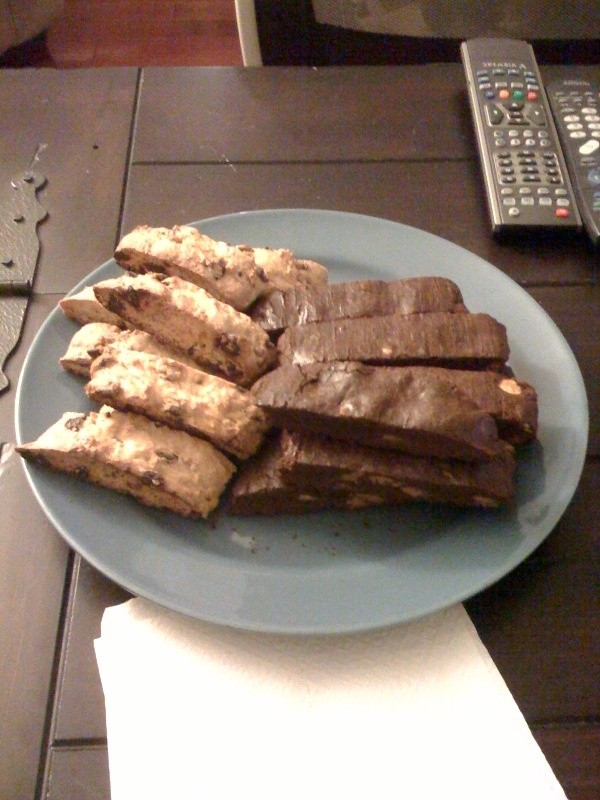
[0,67,600,800]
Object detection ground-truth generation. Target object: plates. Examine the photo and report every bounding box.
[14,210,588,636]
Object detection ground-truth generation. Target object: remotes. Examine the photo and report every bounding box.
[462,36,582,244]
[547,80,600,246]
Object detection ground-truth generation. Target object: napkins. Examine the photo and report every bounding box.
[94,597,570,800]
